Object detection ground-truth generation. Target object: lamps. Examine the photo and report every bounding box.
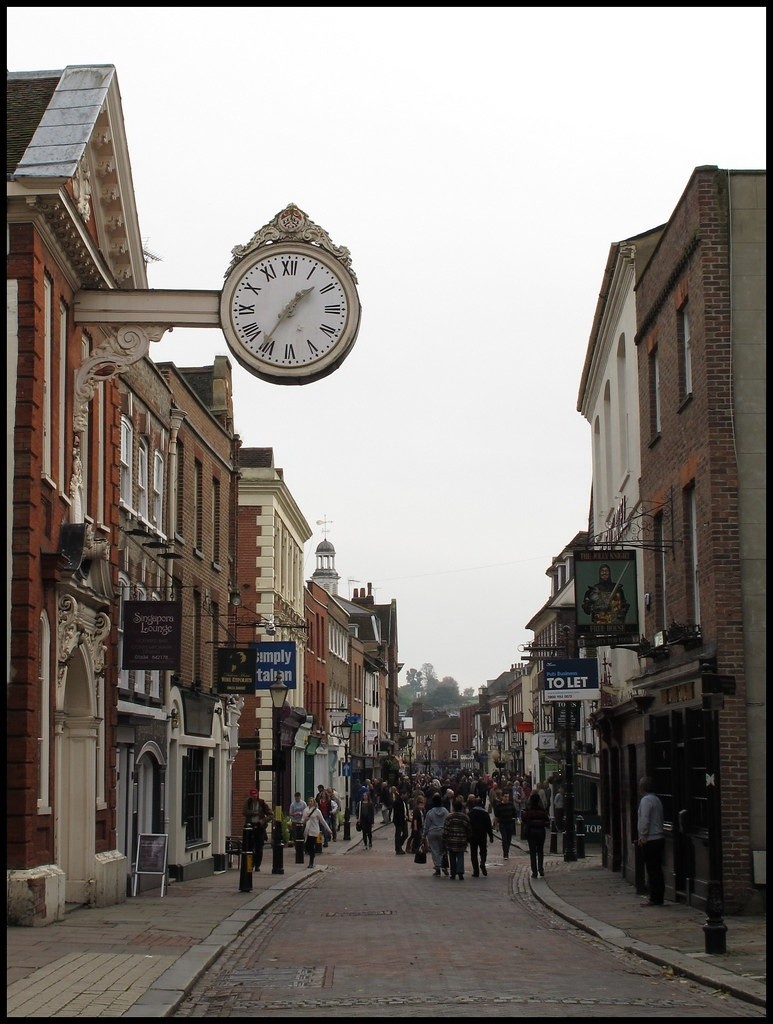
[230,591,244,609]
[125,529,153,538]
[157,552,184,559]
[264,620,276,636]
[142,541,171,549]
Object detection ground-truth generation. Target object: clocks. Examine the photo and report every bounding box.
[229,251,350,369]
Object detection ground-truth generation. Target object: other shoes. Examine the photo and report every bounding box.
[539,870,544,876]
[332,837,336,842]
[459,875,464,880]
[504,853,508,860]
[363,847,367,851]
[480,865,487,876]
[531,873,537,878]
[308,864,314,868]
[396,849,405,854]
[433,868,441,877]
[323,843,328,847]
[442,867,449,875]
[472,872,479,876]
[640,899,664,907]
[255,868,260,871]
[450,874,455,879]
[369,844,372,849]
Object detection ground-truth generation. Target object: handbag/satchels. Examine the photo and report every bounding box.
[441,847,449,868]
[356,821,361,832]
[414,843,426,864]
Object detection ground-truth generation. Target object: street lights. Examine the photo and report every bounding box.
[425,735,433,777]
[423,741,428,774]
[406,730,415,810]
[495,726,506,788]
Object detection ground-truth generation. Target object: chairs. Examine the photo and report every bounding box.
[226,836,243,870]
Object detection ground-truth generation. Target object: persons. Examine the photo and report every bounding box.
[637,777,667,907]
[242,768,566,879]
